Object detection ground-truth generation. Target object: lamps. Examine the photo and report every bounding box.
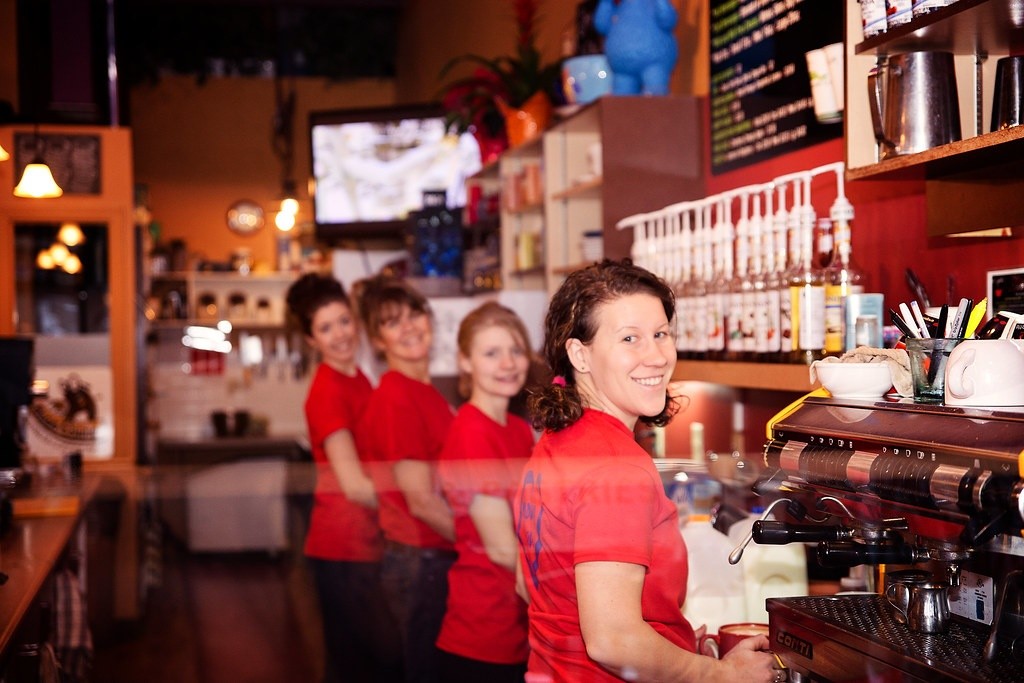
[12,126,66,203]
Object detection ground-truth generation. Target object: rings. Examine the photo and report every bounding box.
[775,670,782,683]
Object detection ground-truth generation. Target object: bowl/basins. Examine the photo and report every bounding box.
[813,361,892,400]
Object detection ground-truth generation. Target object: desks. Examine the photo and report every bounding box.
[0,468,107,654]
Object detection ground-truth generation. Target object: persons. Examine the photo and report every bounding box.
[514,258,786,683]
[357,275,457,682]
[437,302,535,682]
[286,272,377,683]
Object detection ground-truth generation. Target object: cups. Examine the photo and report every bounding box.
[559,53,613,104]
[906,338,964,404]
[855,315,878,347]
[61,453,81,479]
[699,622,769,660]
[945,340,1024,407]
[885,569,952,634]
[990,56,1024,132]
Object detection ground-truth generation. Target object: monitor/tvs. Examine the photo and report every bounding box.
[309,101,482,239]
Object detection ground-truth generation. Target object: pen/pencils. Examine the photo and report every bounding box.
[926,303,948,385]
[910,300,930,339]
[888,309,932,358]
[899,302,921,337]
[956,298,973,345]
[932,298,967,386]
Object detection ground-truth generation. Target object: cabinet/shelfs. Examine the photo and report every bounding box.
[844,0,1024,182]
[461,88,707,295]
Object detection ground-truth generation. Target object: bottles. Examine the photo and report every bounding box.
[671,218,867,360]
[859,0,960,39]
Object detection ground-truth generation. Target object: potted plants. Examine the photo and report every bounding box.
[429,3,566,150]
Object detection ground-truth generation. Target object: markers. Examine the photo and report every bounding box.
[964,296,987,340]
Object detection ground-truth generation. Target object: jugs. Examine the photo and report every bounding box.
[867,52,961,163]
